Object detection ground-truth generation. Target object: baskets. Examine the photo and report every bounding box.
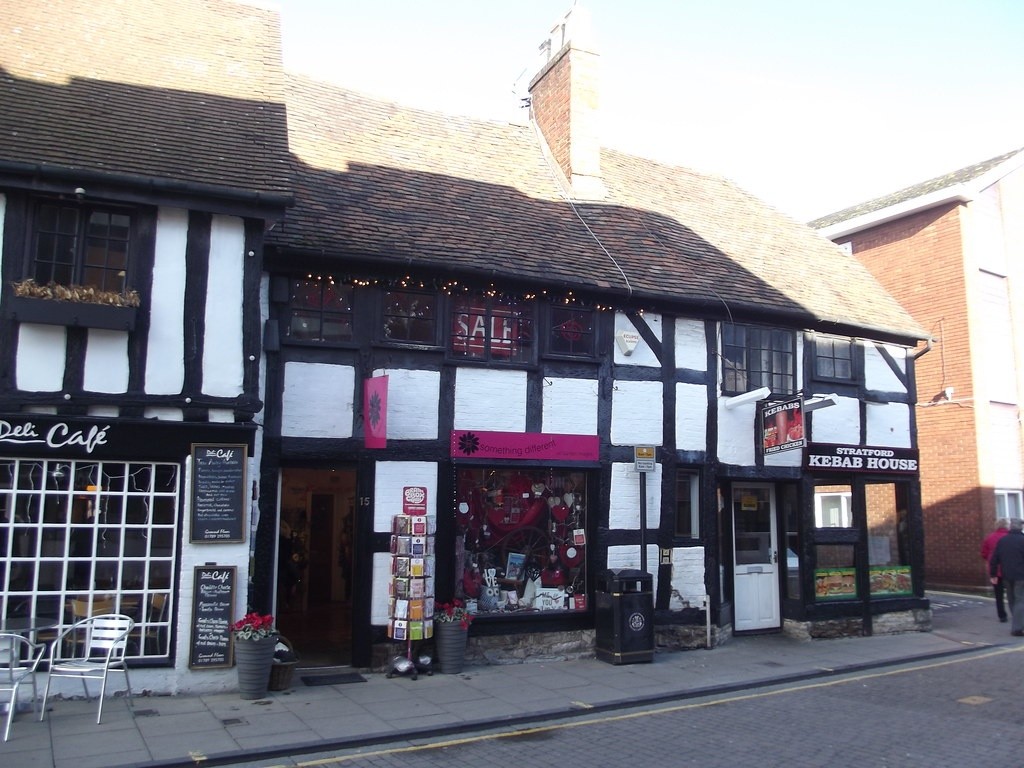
[267,635,302,692]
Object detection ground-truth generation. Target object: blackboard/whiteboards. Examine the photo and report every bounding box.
[191,441,247,544]
[190,562,238,670]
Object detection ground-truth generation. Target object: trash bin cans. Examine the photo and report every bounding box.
[594,569,653,666]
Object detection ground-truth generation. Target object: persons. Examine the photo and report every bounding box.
[989,517,1024,636]
[979,517,1015,621]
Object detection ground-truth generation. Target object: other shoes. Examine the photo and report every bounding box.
[1012,631,1024,637]
[1000,616,1007,622]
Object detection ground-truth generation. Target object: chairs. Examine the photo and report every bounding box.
[0,633,46,742]
[40,614,135,724]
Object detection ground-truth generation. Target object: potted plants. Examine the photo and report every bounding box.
[434,598,475,674]
[229,612,279,699]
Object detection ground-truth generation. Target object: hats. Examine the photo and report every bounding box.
[1010,517,1024,525]
